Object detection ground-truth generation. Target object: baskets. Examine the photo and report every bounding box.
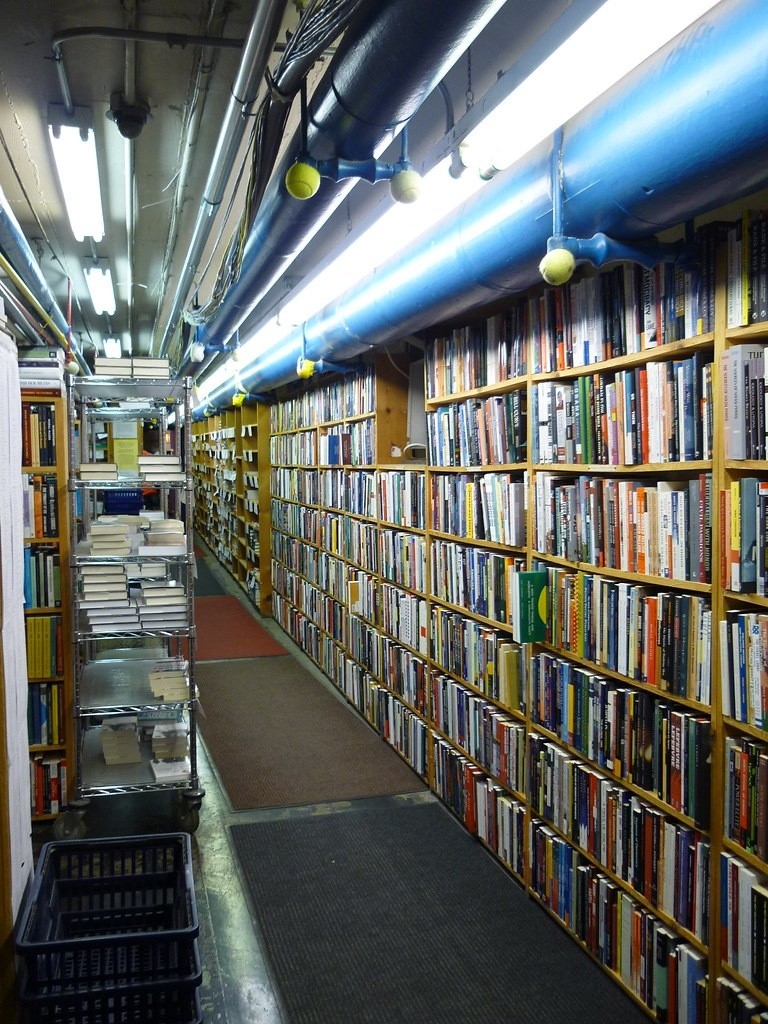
[12,831,206,1024]
[102,487,145,516]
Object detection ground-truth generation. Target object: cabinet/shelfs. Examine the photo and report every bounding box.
[22,227,768,1024]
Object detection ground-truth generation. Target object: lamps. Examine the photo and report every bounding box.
[47,102,106,243]
[102,333,122,359]
[82,257,117,316]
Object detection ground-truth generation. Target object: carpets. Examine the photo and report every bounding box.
[169,595,291,661]
[195,654,428,814]
[224,802,655,1023]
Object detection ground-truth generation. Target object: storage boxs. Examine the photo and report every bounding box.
[14,831,203,1024]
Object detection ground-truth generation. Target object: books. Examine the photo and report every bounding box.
[0,212,768,1024]
